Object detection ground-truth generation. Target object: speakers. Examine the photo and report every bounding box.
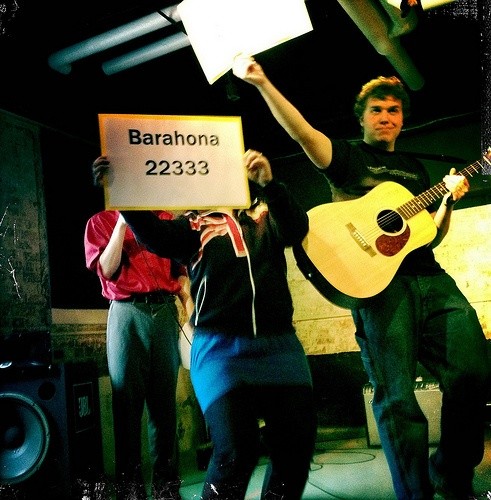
[0,360,109,500]
[361,382,446,449]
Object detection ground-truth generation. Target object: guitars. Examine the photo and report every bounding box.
[291,146,491,310]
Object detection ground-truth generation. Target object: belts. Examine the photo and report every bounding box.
[130,292,176,305]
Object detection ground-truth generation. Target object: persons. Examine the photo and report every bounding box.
[232,52,491,500]
[90,149,318,500]
[84,209,186,500]
